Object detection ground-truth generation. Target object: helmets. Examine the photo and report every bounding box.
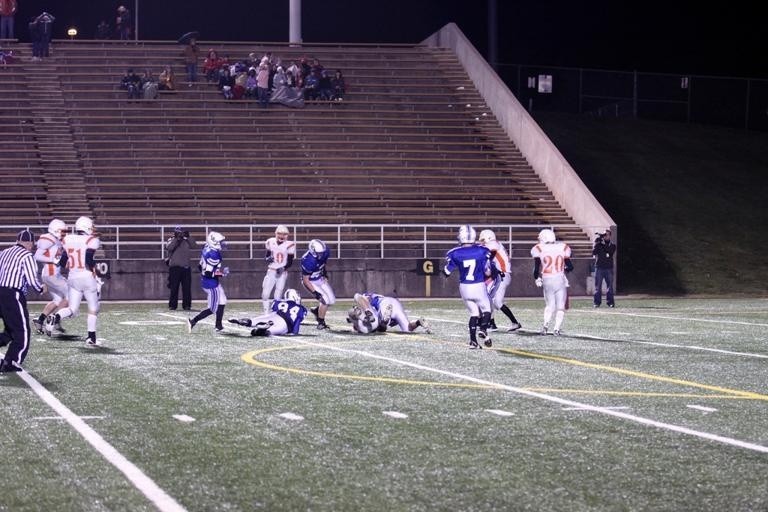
[206,231,225,251]
[275,225,290,243]
[48,216,94,240]
[284,289,301,306]
[537,229,556,244]
[308,239,327,260]
[457,224,497,246]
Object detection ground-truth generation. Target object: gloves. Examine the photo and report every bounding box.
[535,278,544,287]
[276,267,285,275]
[312,290,326,305]
[221,267,231,277]
[266,256,274,264]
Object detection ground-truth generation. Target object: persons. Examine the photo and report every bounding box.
[227,289,308,337]
[47,215,103,349]
[298,239,337,331]
[442,224,496,347]
[0,0,19,45]
[0,231,48,371]
[530,228,573,337]
[477,228,521,332]
[187,230,231,334]
[346,291,431,336]
[555,237,573,310]
[165,227,196,309]
[33,219,69,334]
[111,1,347,112]
[29,12,46,56]
[345,305,399,334]
[591,230,617,307]
[262,225,294,311]
[37,11,56,60]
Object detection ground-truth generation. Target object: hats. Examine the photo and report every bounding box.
[16,230,36,241]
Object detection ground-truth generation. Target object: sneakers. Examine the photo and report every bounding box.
[84,337,103,350]
[418,316,434,334]
[542,326,560,337]
[469,323,522,349]
[311,306,330,330]
[216,318,274,336]
[31,313,65,337]
[363,304,392,326]
[188,317,194,333]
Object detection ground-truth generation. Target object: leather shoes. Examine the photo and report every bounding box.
[0,359,22,374]
[168,307,190,310]
[593,304,614,308]
